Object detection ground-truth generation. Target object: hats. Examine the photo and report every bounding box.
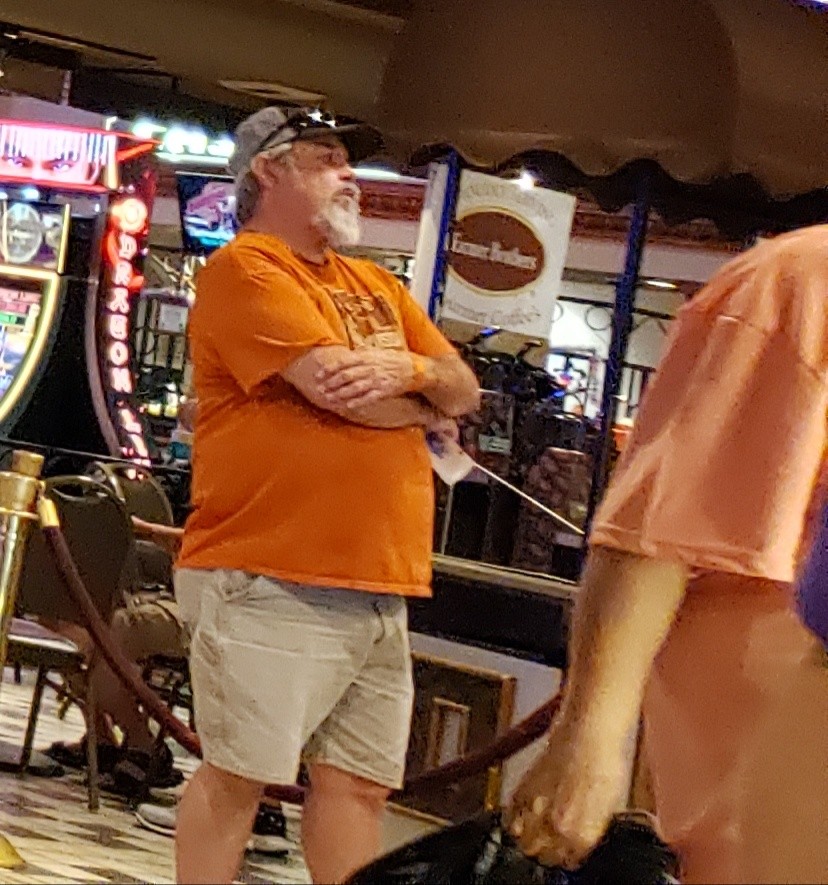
[226,106,383,175]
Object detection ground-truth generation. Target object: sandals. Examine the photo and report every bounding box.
[41,735,120,772]
[96,737,185,795]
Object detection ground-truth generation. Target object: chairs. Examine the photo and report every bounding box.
[0,463,196,813]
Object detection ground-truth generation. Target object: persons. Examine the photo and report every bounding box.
[507,219,827,885]
[42,507,187,796]
[175,102,480,885]
[134,779,294,857]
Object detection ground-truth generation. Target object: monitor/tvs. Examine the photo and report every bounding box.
[174,171,242,256]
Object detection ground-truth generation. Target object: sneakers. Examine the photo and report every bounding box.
[243,801,290,856]
[134,799,255,851]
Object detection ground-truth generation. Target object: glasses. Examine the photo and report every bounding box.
[254,106,361,157]
